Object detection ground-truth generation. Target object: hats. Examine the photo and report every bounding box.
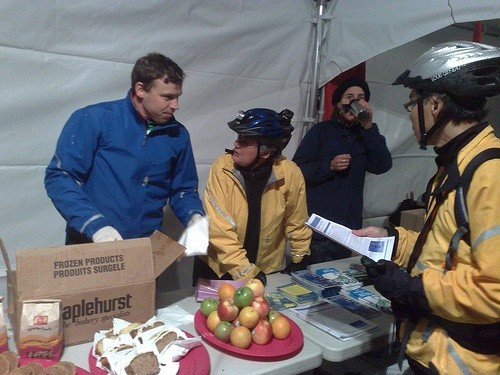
[332,78,370,104]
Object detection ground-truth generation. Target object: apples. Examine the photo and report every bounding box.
[200,278,280,344]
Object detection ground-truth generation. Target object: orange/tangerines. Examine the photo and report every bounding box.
[229,326,251,349]
[272,316,290,339]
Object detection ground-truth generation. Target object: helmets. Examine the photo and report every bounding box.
[391,41,500,97]
[227,108,294,150]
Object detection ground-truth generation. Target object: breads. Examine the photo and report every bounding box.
[95,321,186,375]
[0,351,77,375]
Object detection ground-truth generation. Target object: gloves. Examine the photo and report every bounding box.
[361,255,432,319]
[91,226,123,243]
[188,213,200,225]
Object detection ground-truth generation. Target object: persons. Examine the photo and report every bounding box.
[353,39,500,375]
[193,108,312,288]
[43,53,206,245]
[292,78,393,270]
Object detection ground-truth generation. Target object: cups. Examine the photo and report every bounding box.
[347,98,370,122]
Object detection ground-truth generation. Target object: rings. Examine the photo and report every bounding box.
[343,158,346,162]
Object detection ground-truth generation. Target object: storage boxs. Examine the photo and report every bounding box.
[399,208,426,233]
[0,231,188,348]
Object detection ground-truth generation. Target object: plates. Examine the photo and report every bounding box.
[87,327,211,374]
[193,306,303,358]
[17,356,92,374]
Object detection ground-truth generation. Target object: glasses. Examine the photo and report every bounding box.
[403,93,431,112]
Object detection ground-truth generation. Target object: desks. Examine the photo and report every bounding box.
[260,256,395,362]
[60,292,323,375]
